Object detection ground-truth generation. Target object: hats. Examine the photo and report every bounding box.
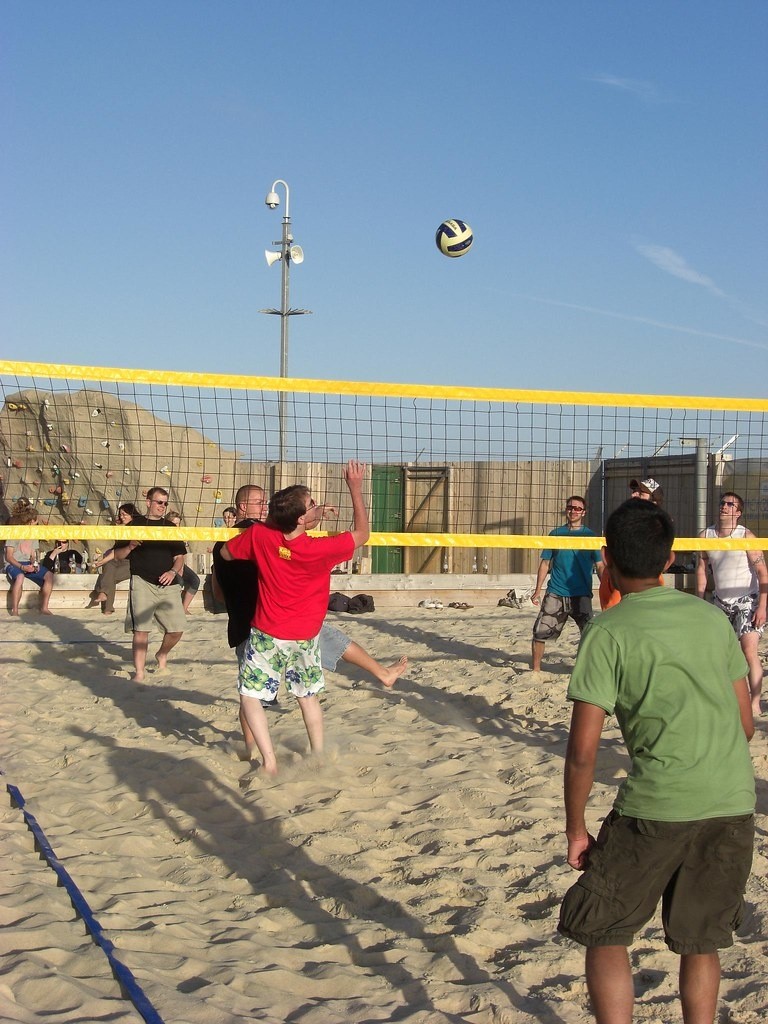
[631,477,663,506]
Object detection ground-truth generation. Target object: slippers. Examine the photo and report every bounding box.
[433,599,444,609]
[419,599,435,609]
[449,602,474,609]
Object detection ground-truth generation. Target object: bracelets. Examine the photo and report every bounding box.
[170,568,177,575]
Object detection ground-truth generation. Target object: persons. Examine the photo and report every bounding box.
[206,459,407,776]
[531,496,604,673]
[0,473,88,615]
[696,492,768,714]
[557,497,754,1024]
[95,487,200,681]
[600,479,662,611]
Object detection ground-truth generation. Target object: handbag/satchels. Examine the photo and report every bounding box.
[329,591,350,611]
[348,593,375,614]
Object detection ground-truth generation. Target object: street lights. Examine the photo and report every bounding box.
[264,176,311,496]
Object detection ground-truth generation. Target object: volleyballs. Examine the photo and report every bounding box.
[436,218,474,259]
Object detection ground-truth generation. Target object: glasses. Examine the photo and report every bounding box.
[150,500,169,507]
[720,500,740,510]
[57,540,69,543]
[567,505,586,511]
